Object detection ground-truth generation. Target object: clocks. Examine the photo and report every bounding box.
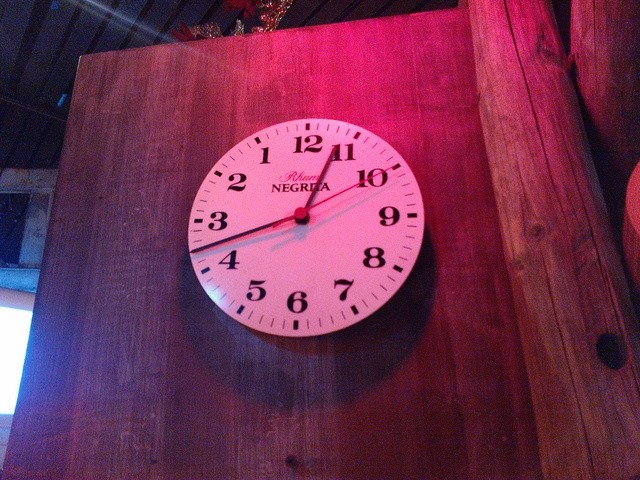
[188,117,426,337]
